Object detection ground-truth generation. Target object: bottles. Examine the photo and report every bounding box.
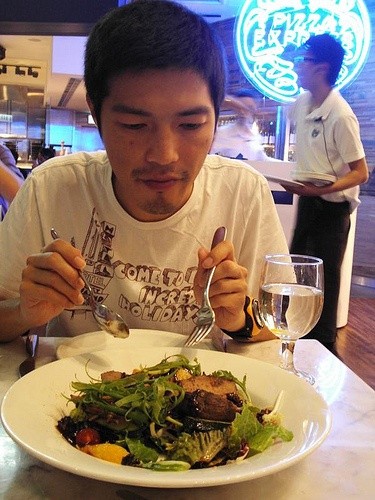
[59,141,64,155]
[290,120,297,144]
[260,120,275,145]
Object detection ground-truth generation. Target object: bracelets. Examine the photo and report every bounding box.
[220,295,264,343]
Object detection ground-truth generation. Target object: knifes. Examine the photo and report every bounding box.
[19,333,39,376]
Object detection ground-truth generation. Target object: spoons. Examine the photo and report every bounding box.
[50,227,129,341]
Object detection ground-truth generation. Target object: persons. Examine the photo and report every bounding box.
[32,148,56,169]
[208,87,271,162]
[279,33,369,361]
[0,144,25,222]
[0,0,298,344]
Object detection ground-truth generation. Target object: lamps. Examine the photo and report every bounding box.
[234,0,371,104]
[88,111,95,124]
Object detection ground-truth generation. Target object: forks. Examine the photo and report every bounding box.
[182,227,227,348]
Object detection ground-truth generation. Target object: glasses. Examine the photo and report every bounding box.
[294,57,327,65]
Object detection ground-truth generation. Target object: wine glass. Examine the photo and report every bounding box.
[258,254,324,385]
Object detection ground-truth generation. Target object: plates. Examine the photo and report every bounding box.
[265,175,305,191]
[54,327,213,360]
[292,170,336,186]
[1,348,332,489]
